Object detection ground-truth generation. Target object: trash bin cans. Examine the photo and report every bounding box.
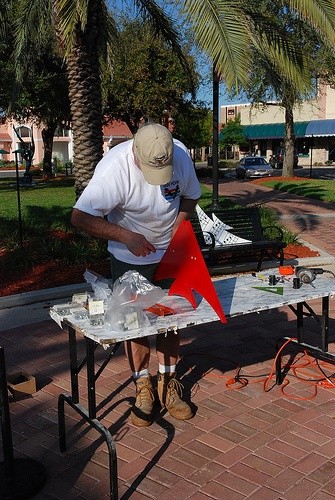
[207,156,213,166]
[51,157,57,174]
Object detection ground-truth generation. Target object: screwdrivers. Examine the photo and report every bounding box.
[148,248,167,251]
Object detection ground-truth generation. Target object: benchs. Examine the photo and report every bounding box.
[189,207,287,271]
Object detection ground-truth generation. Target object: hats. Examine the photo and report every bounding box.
[135,124,175,185]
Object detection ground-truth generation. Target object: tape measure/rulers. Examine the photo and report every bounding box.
[252,287,283,295]
[279,266,295,274]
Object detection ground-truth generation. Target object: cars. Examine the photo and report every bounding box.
[236,156,274,179]
[269,153,298,169]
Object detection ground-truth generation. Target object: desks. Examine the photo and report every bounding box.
[48,258,335,500]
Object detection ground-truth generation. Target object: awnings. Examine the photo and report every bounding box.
[219,119,335,141]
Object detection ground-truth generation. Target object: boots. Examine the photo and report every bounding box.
[156,371,192,420]
[130,376,154,427]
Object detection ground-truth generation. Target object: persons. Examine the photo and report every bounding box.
[70,123,202,428]
